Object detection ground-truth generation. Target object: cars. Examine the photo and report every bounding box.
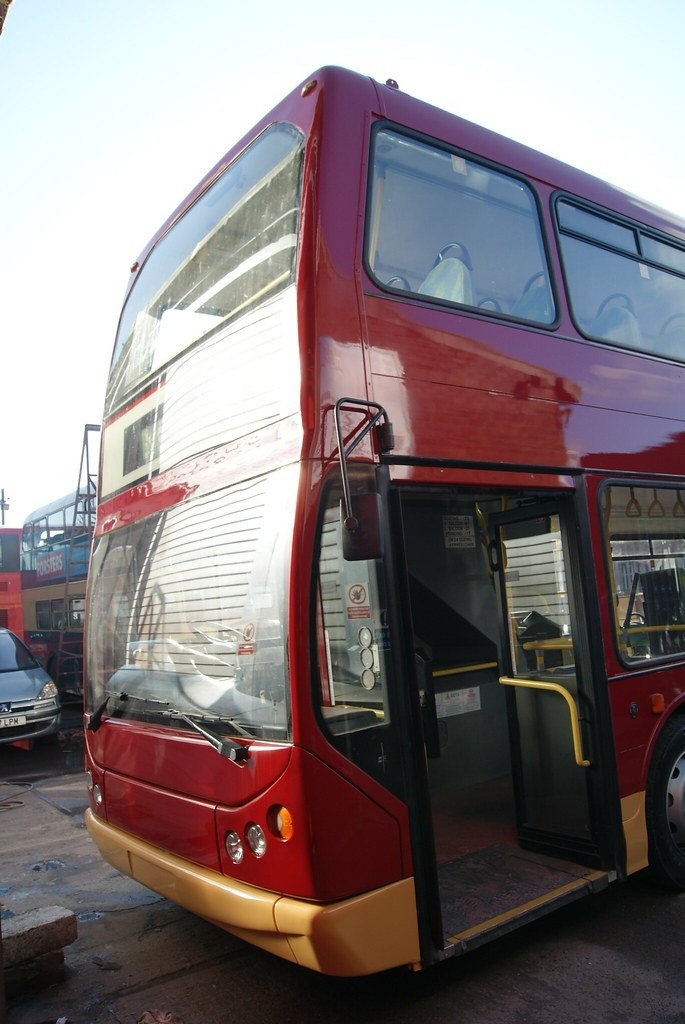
[1,627,61,749]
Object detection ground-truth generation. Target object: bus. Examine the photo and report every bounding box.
[79,66,685,983]
[0,481,98,702]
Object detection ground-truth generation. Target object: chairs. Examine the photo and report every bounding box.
[385,276,412,290]
[477,298,501,312]
[416,239,476,307]
[622,612,647,655]
[652,314,685,358]
[584,290,646,345]
[511,265,552,323]
